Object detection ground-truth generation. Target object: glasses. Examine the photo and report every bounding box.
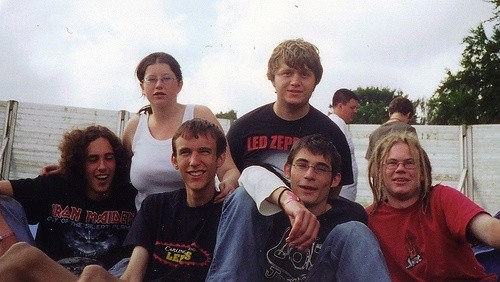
[142,75,179,84]
[383,160,420,169]
[290,161,334,176]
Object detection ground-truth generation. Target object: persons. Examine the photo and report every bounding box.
[363,96,419,161]
[0,118,230,282]
[227,39,354,203]
[40,52,240,213]
[329,89,358,202]
[0,125,137,282]
[204,134,391,282]
[363,132,500,282]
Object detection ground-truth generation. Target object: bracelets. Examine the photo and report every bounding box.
[278,187,294,211]
[283,191,305,205]
